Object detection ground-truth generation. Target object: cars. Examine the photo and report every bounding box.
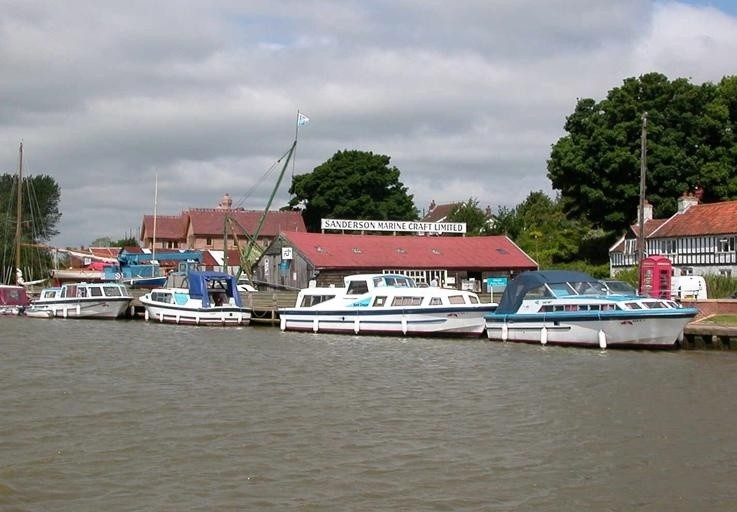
[582,280,639,296]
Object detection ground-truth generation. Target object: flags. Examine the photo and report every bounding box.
[298,113,310,127]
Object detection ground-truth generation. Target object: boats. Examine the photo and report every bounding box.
[483,271,699,350]
[277,274,500,340]
[26,270,255,327]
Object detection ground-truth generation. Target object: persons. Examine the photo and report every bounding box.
[430,275,439,287]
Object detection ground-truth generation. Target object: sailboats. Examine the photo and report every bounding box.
[0,140,57,316]
